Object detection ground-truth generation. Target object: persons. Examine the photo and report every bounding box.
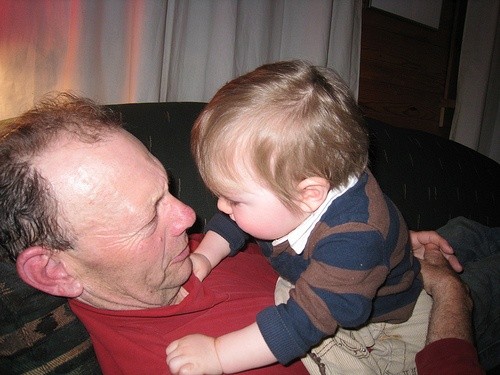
[167,63,432,374]
[0,90,495,373]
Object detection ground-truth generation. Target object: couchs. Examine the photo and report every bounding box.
[0,101,500,375]
